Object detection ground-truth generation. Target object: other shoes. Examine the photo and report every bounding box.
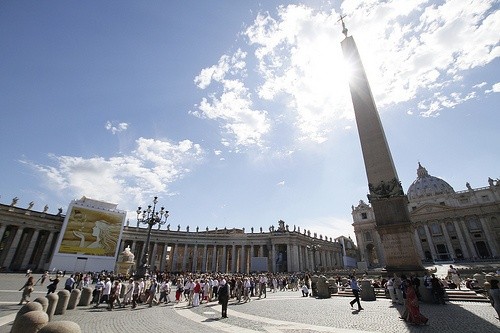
[359,308,364,310]
[350,302,353,307]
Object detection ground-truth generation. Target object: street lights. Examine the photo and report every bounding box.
[136,195,169,279]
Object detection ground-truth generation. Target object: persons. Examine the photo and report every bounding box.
[19,264,500,319]
[349,276,364,310]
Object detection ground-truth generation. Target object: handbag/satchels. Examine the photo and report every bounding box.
[424,280,429,287]
[179,287,183,292]
